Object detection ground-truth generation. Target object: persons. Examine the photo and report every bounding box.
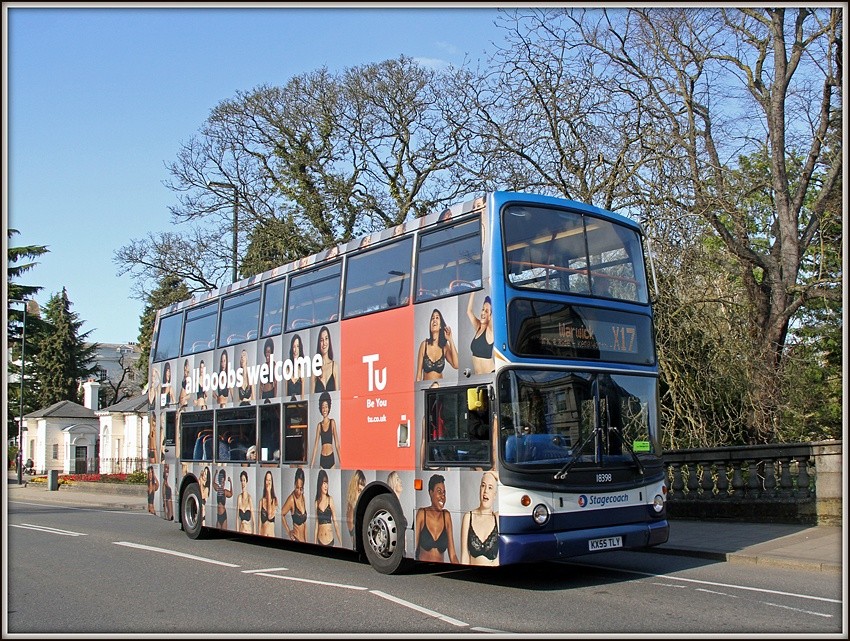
[162,464,173,521]
[462,390,536,450]
[461,471,500,567]
[238,326,338,406]
[314,470,341,547]
[387,471,402,497]
[282,468,307,542]
[148,368,159,514]
[246,445,256,460]
[416,474,459,564]
[346,470,366,550]
[593,272,613,298]
[416,292,495,389]
[257,471,279,537]
[214,469,232,530]
[199,467,210,526]
[236,471,256,534]
[161,349,233,463]
[311,392,341,469]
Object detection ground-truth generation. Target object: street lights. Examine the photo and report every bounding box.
[8,299,27,485]
[206,181,238,284]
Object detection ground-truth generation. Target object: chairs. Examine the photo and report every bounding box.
[190,280,475,356]
[191,427,250,462]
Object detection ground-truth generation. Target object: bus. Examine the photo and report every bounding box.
[147,191,670,575]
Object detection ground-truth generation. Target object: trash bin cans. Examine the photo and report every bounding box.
[48,469,58,491]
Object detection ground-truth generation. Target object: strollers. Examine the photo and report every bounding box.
[22,459,36,476]
